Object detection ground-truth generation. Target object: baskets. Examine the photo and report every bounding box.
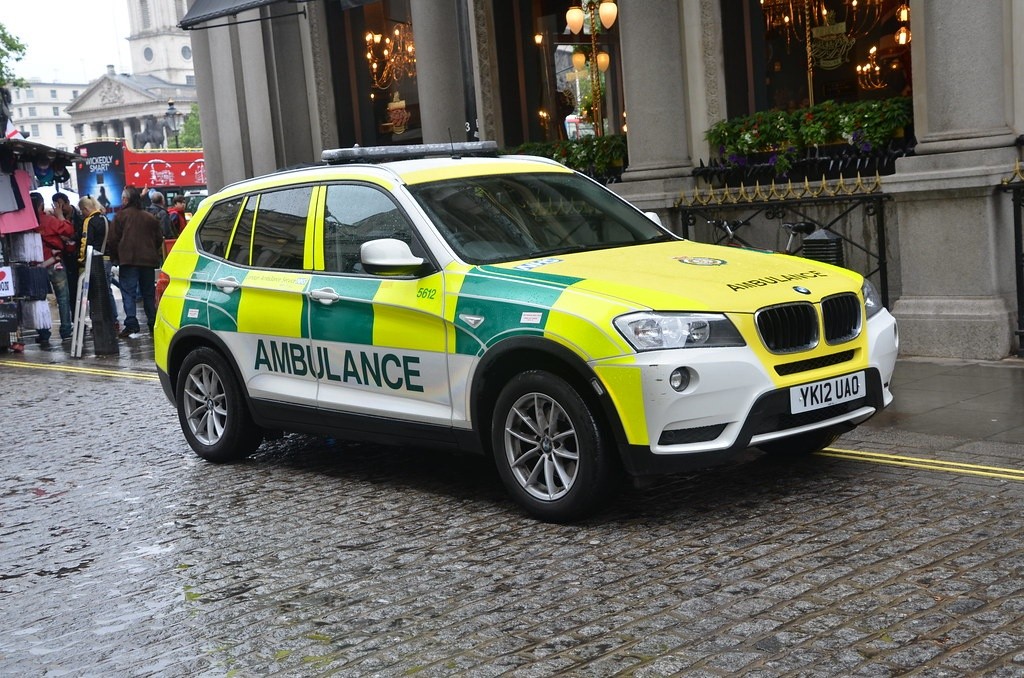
[802,237,844,268]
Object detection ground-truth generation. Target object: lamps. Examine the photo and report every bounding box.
[538,111,550,130]
[362,18,416,90]
[534,32,543,44]
[759,0,912,92]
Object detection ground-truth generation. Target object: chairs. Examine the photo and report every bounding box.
[334,209,366,272]
[444,195,503,247]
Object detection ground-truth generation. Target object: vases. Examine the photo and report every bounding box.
[611,159,625,167]
[742,137,904,164]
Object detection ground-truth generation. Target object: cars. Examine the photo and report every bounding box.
[183,189,209,219]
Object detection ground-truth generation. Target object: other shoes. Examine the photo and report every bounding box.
[149,327,154,334]
[114,323,119,330]
[35,332,51,343]
[62,336,72,344]
[119,327,140,338]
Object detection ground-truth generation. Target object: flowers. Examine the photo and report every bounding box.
[497,134,628,175]
[702,99,908,179]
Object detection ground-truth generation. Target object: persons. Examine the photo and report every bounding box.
[110,185,163,339]
[73,195,120,332]
[52,193,87,332]
[29,192,75,343]
[140,184,178,240]
[168,195,186,233]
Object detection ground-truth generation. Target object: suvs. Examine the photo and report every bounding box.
[150,139,901,525]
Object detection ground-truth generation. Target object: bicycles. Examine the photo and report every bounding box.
[706,212,845,270]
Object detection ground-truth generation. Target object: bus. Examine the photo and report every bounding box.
[69,138,205,221]
